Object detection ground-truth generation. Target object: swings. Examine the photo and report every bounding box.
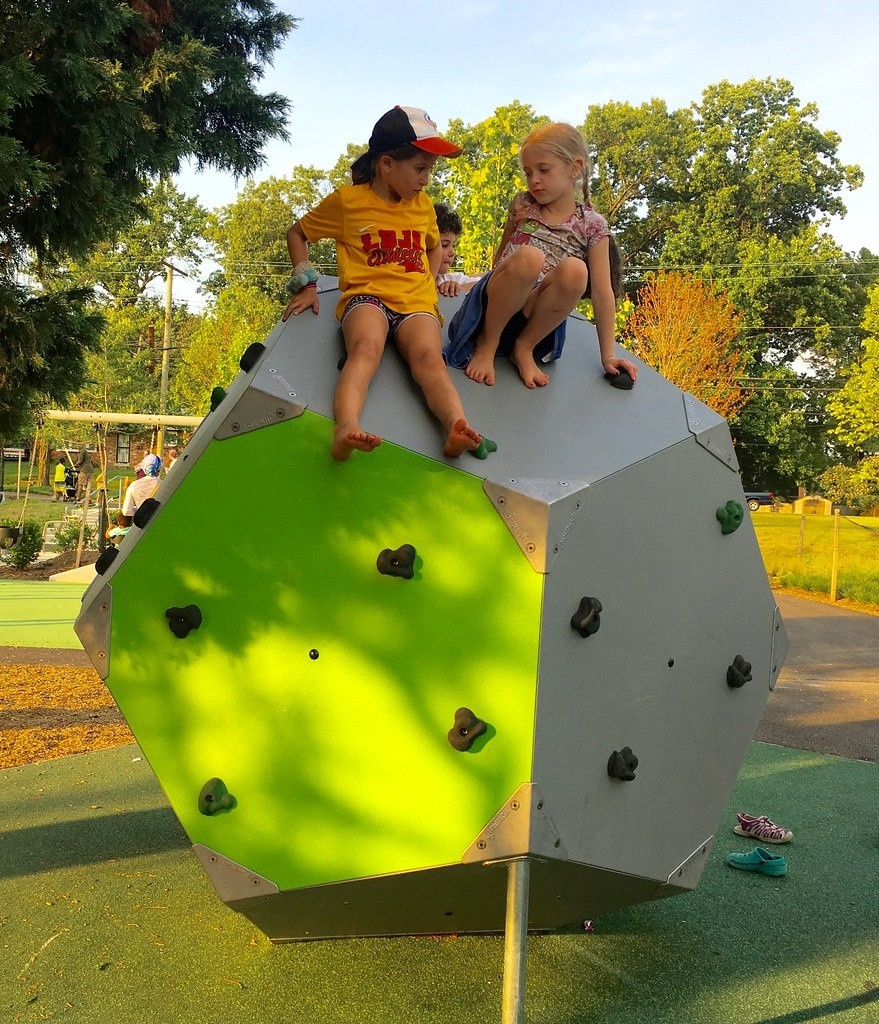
[0,422,42,550]
[94,422,160,549]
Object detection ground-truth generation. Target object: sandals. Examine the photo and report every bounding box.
[727,847,788,875]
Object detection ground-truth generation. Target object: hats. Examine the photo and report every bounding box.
[142,454,161,474]
[169,450,177,457]
[368,105,463,160]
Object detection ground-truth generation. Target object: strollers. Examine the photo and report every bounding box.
[63,465,81,501]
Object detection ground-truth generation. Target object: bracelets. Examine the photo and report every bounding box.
[285,259,319,295]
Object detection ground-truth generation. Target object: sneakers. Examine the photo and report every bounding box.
[734,813,793,844]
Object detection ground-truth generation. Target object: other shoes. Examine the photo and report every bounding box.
[88,499,93,505]
[76,497,80,503]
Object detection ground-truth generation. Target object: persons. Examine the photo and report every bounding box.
[434,204,489,298]
[105,448,180,550]
[282,105,483,462]
[71,442,93,504]
[442,122,640,389]
[52,458,69,502]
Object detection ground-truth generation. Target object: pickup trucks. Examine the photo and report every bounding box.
[744,492,774,511]
